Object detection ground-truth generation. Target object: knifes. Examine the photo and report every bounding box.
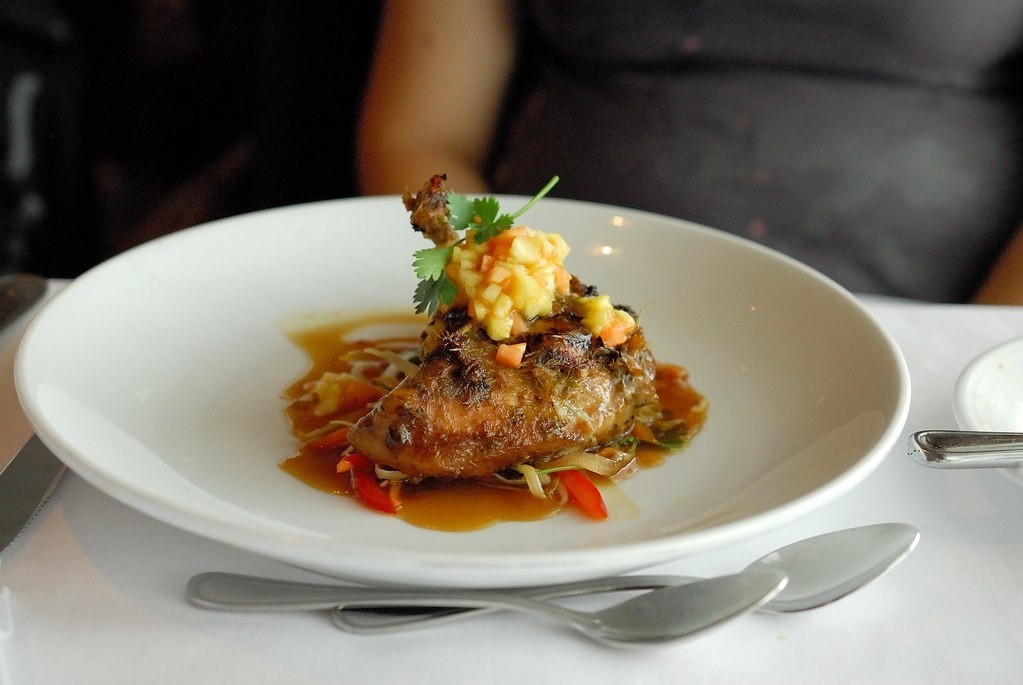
[0,420,67,552]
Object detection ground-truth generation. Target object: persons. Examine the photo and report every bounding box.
[23,0,1023,306]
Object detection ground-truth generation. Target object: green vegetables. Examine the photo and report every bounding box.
[411,176,559,317]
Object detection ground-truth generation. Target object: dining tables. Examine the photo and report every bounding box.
[0,280,1023,685]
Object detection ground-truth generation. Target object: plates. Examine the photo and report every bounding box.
[14,194,912,589]
[953,338,1023,487]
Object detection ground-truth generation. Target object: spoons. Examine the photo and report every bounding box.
[333,523,921,635]
[187,572,789,651]
